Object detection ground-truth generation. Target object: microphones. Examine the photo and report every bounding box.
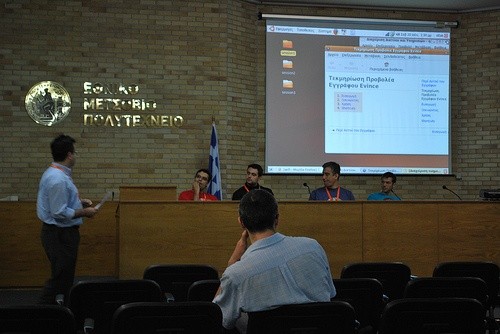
[442,186,461,200]
[391,189,401,200]
[303,183,312,200]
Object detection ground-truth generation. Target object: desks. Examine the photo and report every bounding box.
[115,200,500,280]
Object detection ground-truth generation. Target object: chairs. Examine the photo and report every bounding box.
[1,261,500,334]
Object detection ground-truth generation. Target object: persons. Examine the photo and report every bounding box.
[178,168,218,201]
[36,135,98,305]
[308,162,356,200]
[212,189,337,334]
[232,163,274,200]
[367,172,400,201]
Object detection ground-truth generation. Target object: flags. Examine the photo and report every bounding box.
[207,121,222,201]
